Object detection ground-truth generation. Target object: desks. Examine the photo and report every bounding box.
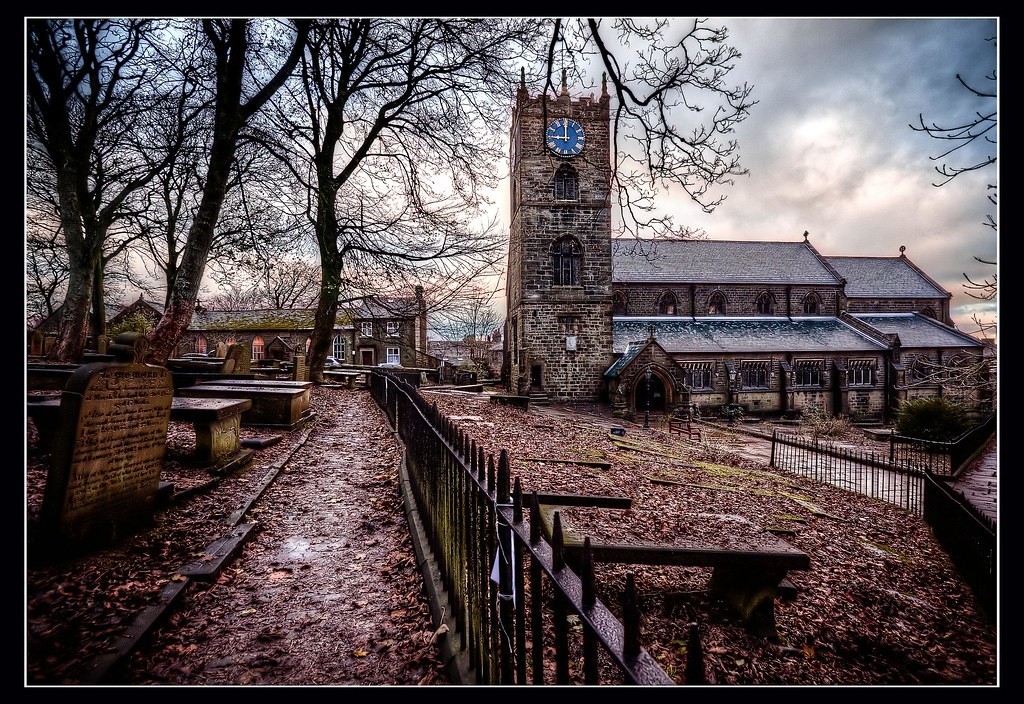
[163,396,255,478]
[537,504,809,624]
[322,369,371,387]
[250,367,281,379]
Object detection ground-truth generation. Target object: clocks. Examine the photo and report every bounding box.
[546,117,584,158]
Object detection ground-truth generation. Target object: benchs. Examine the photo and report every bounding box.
[668,416,702,442]
